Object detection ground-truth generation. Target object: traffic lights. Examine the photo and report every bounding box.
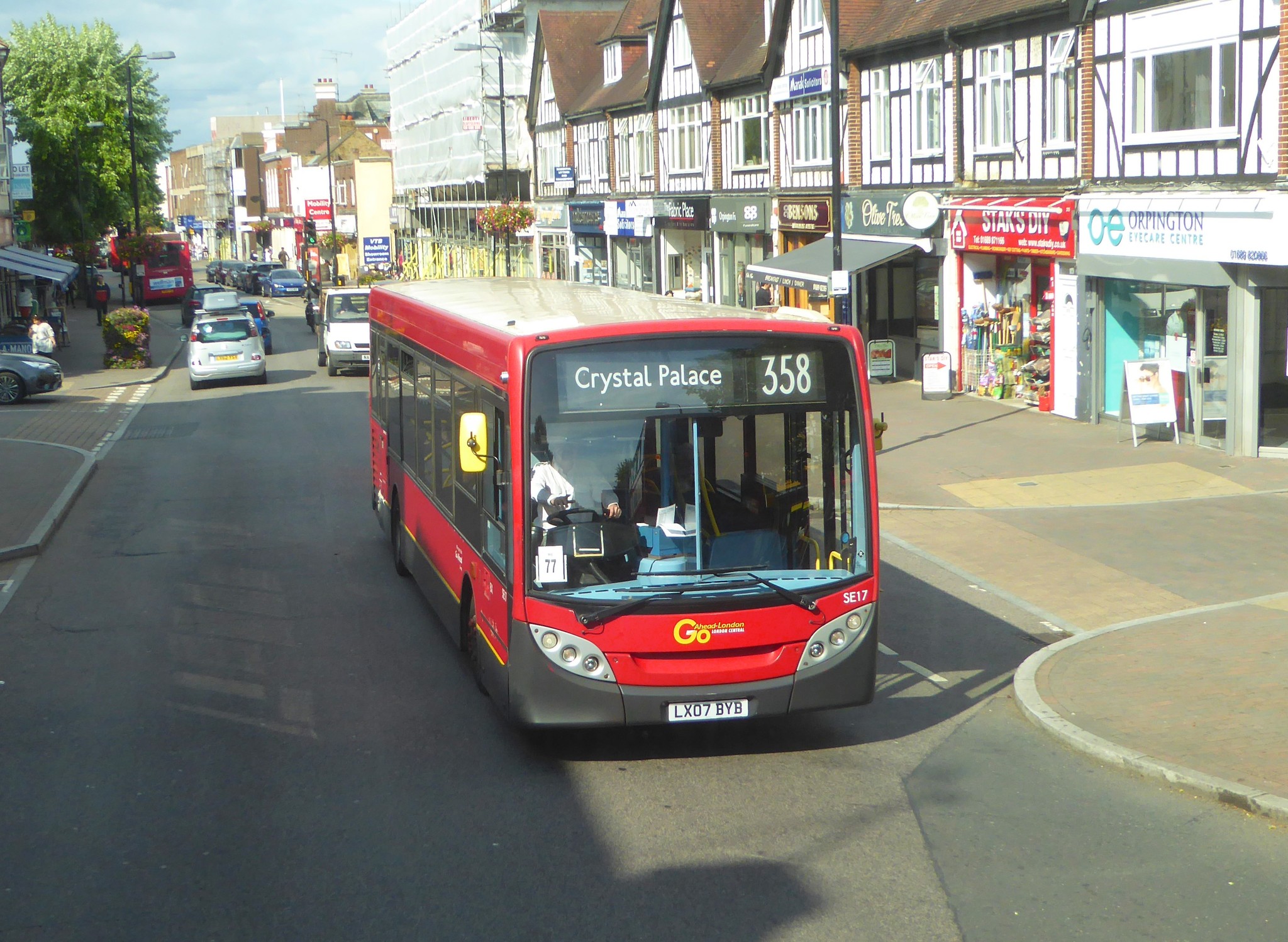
[186,233,191,239]
[216,232,223,239]
[303,221,316,244]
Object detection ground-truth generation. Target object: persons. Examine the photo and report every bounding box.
[303,280,321,325]
[388,262,397,277]
[1202,360,1227,406]
[191,241,208,259]
[129,257,146,309]
[92,274,111,326]
[1166,302,1190,337]
[671,428,695,498]
[738,268,774,306]
[1139,363,1170,406]
[324,255,338,286]
[28,314,57,357]
[335,296,360,316]
[530,442,622,530]
[249,245,289,269]
[665,290,674,297]
[397,249,405,276]
[17,283,39,325]
[35,275,76,308]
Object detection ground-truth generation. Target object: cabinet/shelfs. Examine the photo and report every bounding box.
[1022,309,1051,405]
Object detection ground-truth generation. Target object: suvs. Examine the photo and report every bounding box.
[174,282,228,329]
[180,306,267,390]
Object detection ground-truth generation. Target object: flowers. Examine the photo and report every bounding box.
[249,220,275,234]
[316,231,346,251]
[475,194,536,237]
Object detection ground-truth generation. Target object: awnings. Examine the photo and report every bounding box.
[0,245,80,292]
[745,237,916,295]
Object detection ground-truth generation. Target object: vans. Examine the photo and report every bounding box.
[313,288,373,377]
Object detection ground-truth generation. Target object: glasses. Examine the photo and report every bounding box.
[1139,372,1155,382]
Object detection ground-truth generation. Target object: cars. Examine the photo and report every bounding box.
[0,351,64,405]
[48,234,110,269]
[205,259,308,299]
[239,299,275,355]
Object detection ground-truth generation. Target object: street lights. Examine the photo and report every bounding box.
[127,51,175,234]
[74,121,104,308]
[454,42,511,278]
[299,117,339,287]
[0,44,17,244]
[239,145,267,253]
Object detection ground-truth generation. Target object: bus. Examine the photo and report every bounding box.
[125,239,195,306]
[367,277,889,735]
[108,235,130,275]
